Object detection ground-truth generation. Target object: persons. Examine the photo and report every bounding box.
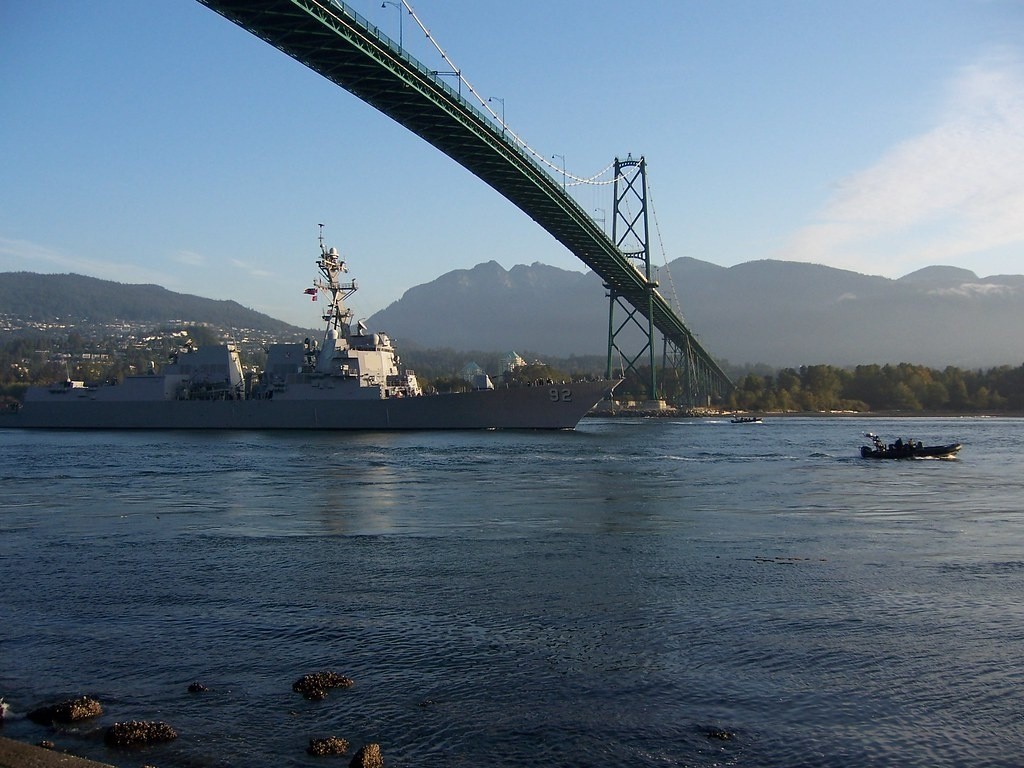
[908,439,913,447]
[527,377,553,385]
[895,437,903,448]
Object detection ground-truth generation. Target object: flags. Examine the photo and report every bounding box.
[312,296,317,301]
[303,288,317,295]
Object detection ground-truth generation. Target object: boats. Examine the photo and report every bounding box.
[729,416,764,423]
[861,432,961,463]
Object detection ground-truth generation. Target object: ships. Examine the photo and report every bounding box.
[0,223,626,433]
